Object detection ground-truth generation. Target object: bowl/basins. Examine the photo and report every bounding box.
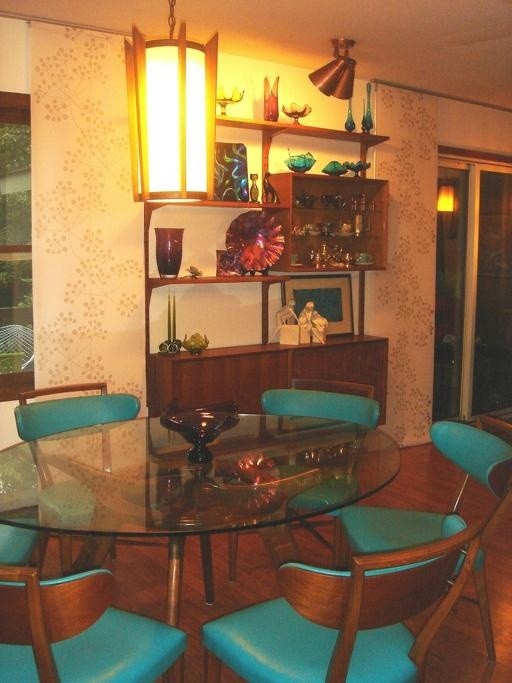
[283,157,317,173]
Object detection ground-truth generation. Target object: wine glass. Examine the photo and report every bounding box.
[214,81,246,116]
[162,463,240,527]
[280,102,313,125]
[160,407,242,464]
[343,159,372,177]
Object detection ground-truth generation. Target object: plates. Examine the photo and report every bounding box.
[212,141,250,202]
[320,159,348,176]
[224,207,285,272]
[228,452,280,513]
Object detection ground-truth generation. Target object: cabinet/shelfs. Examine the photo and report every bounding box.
[144,115,390,426]
[145,416,368,532]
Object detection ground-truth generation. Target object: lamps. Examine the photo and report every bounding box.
[308,36,358,100]
[124,0,219,202]
[437,169,456,212]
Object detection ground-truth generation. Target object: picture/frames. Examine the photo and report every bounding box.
[281,273,354,337]
